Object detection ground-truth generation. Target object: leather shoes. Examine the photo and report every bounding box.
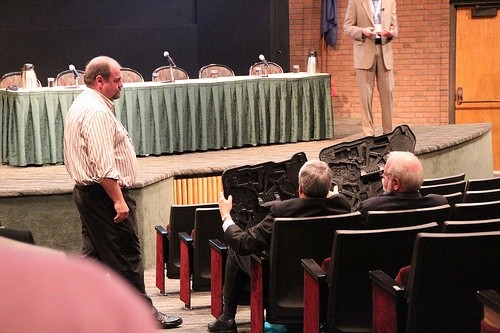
[208,318,238,333]
[158,311,182,329]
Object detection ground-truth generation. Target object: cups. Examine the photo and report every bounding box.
[293,64,300,76]
[152,72,160,83]
[47,77,57,88]
[256,67,262,77]
[374,23,383,33]
[211,69,218,80]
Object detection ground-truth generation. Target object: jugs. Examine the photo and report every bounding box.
[307,51,317,75]
[23,63,38,91]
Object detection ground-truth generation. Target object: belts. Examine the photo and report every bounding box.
[375,38,381,44]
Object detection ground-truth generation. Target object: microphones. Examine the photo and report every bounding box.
[259,54,270,67]
[68,64,80,77]
[163,51,176,67]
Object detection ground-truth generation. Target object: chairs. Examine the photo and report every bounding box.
[154,172,500,333]
[0,61,286,90]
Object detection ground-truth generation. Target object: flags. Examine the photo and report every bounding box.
[320,0,338,47]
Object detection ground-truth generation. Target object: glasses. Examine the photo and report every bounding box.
[379,170,391,177]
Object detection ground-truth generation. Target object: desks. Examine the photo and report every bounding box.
[0,71,333,166]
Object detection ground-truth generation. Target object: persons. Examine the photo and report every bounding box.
[64,56,183,328]
[207,161,350,333]
[343,0,398,136]
[357,150,449,225]
